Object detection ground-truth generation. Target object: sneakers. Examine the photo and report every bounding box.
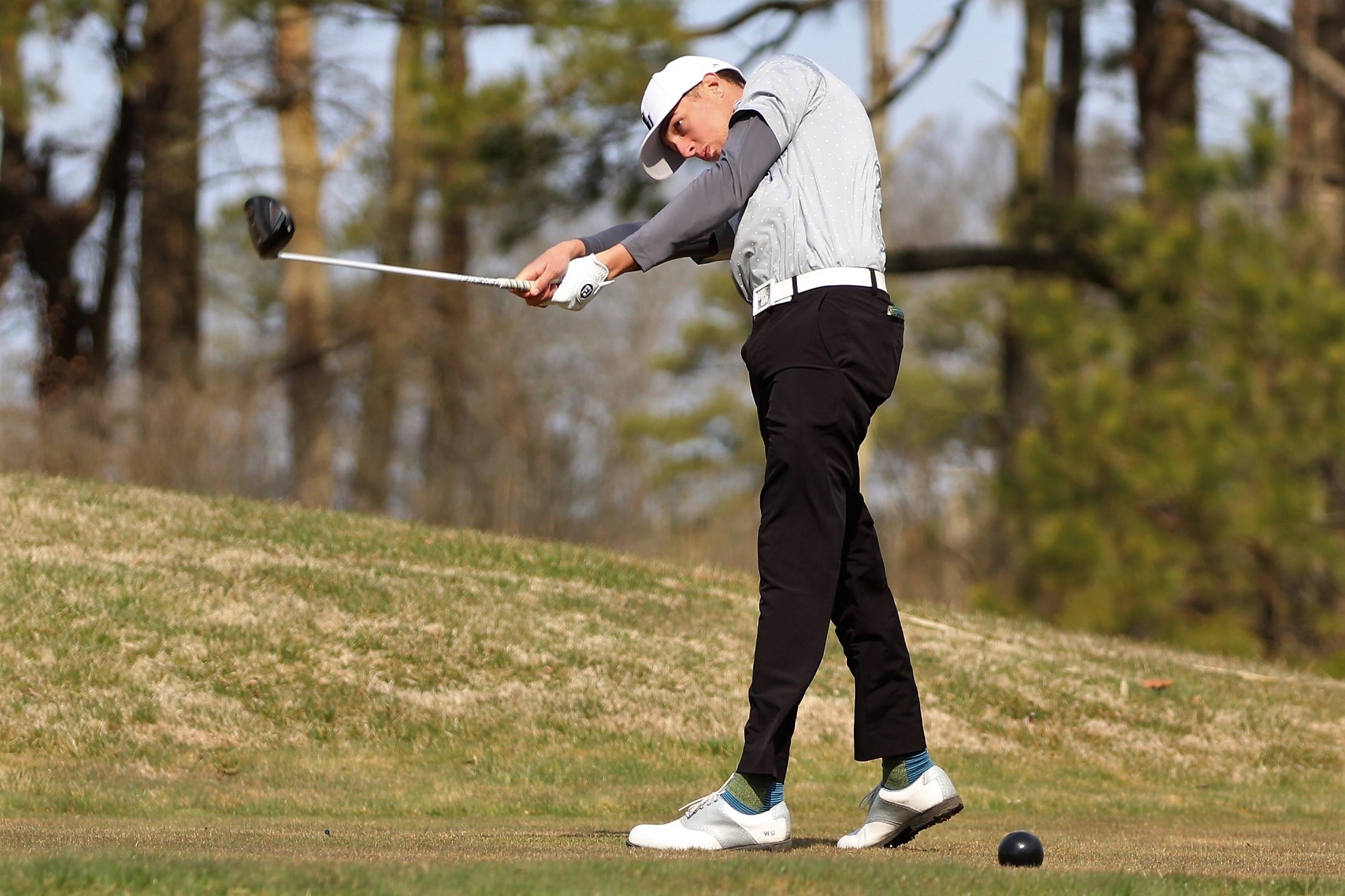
[627,772,793,851]
[835,765,963,849]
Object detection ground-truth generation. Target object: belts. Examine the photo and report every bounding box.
[753,267,888,317]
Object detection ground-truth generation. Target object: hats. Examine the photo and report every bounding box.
[640,55,743,181]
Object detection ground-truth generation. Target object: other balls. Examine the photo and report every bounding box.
[996,830,1046,871]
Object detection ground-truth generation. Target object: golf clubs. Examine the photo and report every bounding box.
[243,196,557,293]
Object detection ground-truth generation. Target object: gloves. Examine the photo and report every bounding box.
[545,253,615,312]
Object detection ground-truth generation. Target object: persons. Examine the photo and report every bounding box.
[510,54,964,857]
